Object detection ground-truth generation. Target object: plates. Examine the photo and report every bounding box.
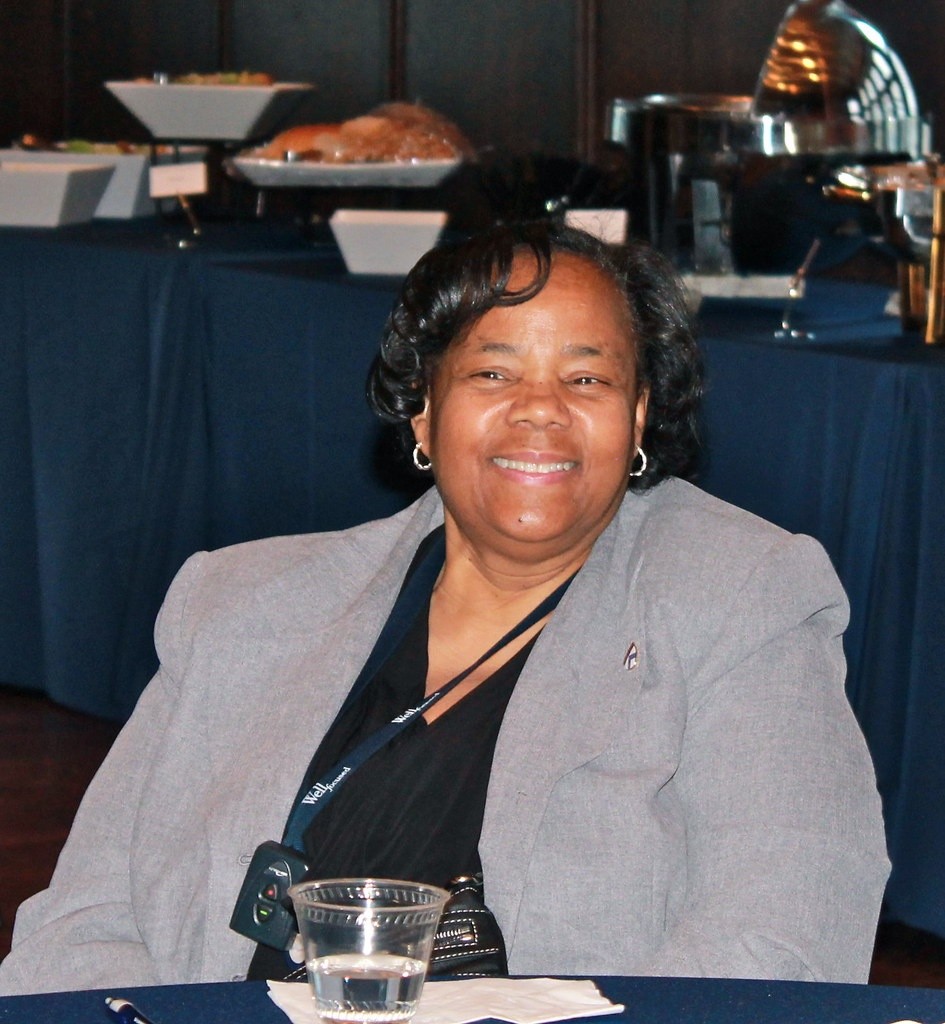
[234,156,462,186]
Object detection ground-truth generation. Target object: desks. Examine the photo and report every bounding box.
[2,207,945,944]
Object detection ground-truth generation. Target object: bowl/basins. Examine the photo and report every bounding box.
[0,161,116,229]
[0,140,210,219]
[106,80,315,141]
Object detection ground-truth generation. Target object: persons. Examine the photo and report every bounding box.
[0,220,892,997]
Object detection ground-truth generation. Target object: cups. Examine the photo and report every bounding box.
[288,878,450,1023]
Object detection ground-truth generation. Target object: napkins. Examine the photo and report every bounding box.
[267,975,624,1024]
